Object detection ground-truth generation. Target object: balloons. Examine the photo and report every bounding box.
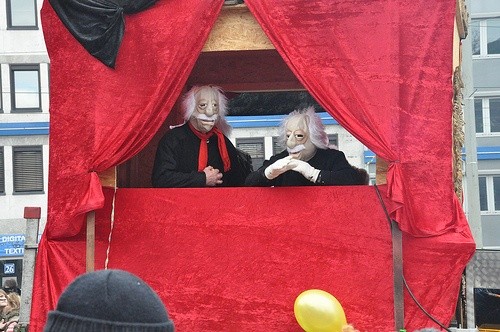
[293,289,348,332]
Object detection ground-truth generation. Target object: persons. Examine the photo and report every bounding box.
[151,85,254,187]
[43,269,175,332]
[245,106,369,187]
[0,278,22,332]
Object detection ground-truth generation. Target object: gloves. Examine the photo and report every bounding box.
[290,159,321,183]
[265,153,301,180]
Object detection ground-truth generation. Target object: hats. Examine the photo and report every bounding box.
[43,269,175,332]
